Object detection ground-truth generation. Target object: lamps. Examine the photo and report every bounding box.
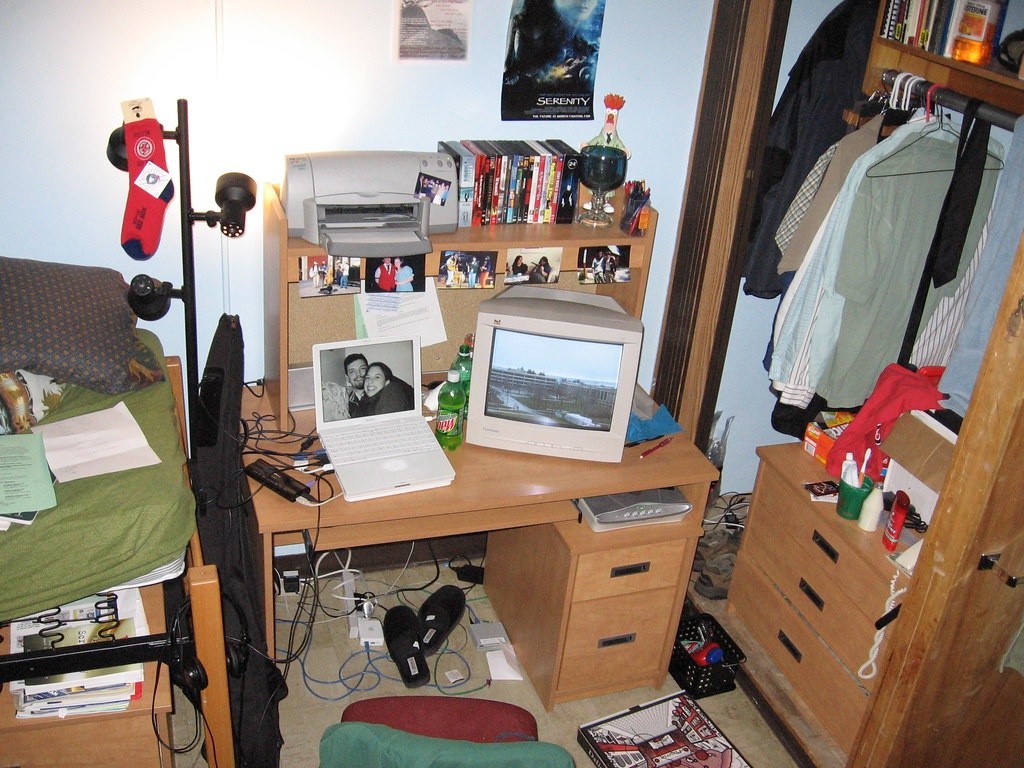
[105,99,257,485]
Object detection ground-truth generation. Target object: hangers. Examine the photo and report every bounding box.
[797,67,1007,186]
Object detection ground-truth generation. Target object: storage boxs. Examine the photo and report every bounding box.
[956,0,1001,42]
[668,611,747,699]
[576,689,754,768]
[880,406,959,523]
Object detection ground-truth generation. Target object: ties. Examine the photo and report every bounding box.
[387,265,389,272]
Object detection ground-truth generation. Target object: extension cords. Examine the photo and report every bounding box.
[342,572,358,640]
[277,578,302,596]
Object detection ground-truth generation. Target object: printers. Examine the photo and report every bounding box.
[282,151,459,259]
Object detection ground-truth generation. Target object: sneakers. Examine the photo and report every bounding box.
[694,553,738,601]
[691,530,739,572]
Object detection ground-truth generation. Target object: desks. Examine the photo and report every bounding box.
[0,583,173,768]
[238,379,720,714]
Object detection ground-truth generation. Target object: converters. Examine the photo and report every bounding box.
[457,564,485,584]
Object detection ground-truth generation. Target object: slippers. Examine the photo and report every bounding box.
[417,585,466,656]
[383,605,430,686]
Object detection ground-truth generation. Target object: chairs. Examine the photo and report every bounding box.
[318,695,578,767]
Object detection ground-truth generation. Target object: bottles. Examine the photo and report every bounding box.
[952,0,1002,66]
[433,369,466,451]
[856,482,884,532]
[451,333,477,421]
[679,640,723,666]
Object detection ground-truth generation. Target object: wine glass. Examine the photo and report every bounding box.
[578,144,627,229]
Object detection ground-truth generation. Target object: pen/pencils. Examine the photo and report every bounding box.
[621,180,650,236]
[625,435,664,447]
[639,437,673,458]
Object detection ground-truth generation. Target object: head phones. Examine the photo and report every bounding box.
[169,591,251,696]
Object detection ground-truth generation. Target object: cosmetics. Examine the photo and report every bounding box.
[883,490,911,552]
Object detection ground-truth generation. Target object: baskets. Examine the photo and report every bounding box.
[668,611,746,699]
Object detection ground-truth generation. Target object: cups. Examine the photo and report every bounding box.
[836,473,874,520]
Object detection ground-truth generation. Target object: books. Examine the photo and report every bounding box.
[879,0,1008,60]
[0,434,57,530]
[7,602,145,717]
[437,139,581,224]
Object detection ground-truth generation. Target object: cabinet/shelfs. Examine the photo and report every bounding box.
[723,441,931,759]
[259,178,660,439]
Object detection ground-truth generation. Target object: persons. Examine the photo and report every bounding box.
[414,175,449,206]
[445,252,492,288]
[592,251,617,283]
[392,256,415,291]
[530,256,551,281]
[374,256,397,292]
[310,259,350,290]
[511,255,529,276]
[342,351,415,418]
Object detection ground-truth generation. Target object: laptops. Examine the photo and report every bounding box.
[312,335,456,497]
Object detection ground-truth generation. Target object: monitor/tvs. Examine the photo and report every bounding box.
[465,285,644,464]
